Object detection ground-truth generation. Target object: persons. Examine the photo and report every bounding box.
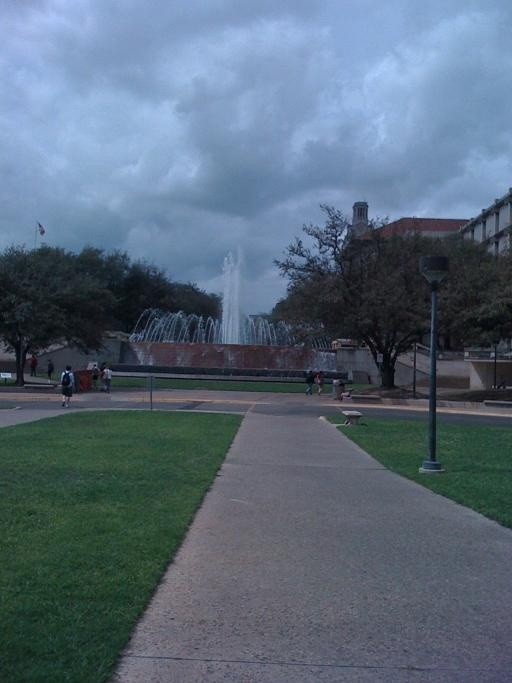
[46,360,54,384]
[29,355,38,377]
[61,365,75,408]
[306,370,315,396]
[90,363,112,394]
[316,371,323,396]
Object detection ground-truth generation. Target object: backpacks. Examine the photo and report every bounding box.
[62,370,71,385]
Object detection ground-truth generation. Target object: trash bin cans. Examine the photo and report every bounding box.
[73,370,95,392]
[333,379,345,401]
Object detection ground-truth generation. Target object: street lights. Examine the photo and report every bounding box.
[493,339,500,388]
[418,253,452,475]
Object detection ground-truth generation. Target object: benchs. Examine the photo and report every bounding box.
[342,410,363,426]
[350,394,380,403]
[483,400,512,405]
[23,384,57,391]
